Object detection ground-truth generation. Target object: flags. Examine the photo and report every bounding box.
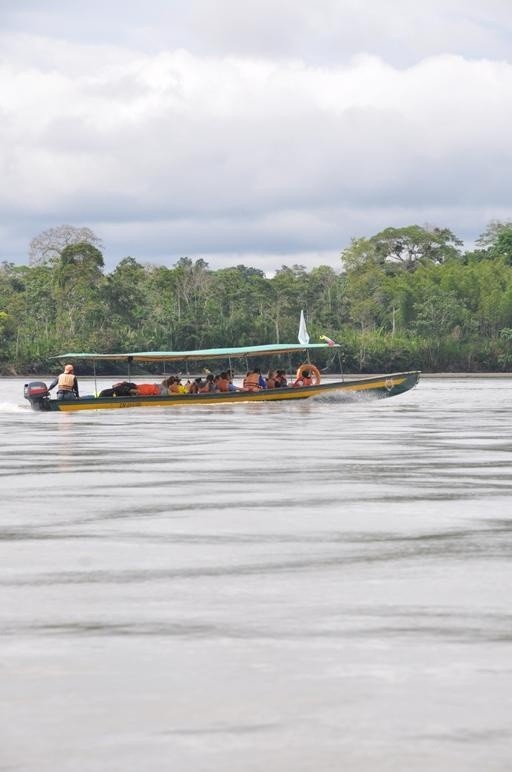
[297,310,311,346]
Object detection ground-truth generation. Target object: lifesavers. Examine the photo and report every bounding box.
[297,364,321,384]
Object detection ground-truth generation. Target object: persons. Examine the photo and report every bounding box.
[103,364,313,397]
[48,364,82,401]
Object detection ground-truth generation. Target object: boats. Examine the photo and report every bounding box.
[24,309,421,411]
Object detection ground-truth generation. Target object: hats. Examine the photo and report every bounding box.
[64,365,74,374]
[175,374,182,380]
